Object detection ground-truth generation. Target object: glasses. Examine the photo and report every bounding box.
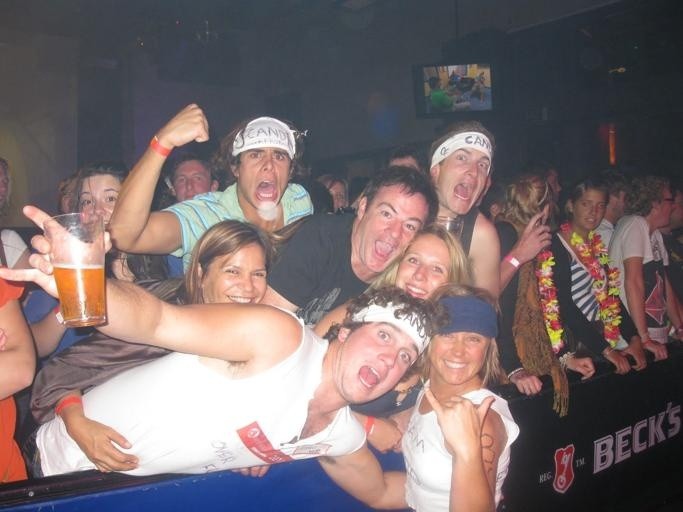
[660,198,675,203]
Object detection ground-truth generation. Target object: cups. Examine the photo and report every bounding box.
[41,211,109,327]
[534,218,551,253]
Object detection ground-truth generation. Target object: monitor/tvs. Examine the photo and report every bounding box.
[411,58,503,120]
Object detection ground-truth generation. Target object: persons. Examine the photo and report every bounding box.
[1,104,681,511]
[428,76,472,113]
